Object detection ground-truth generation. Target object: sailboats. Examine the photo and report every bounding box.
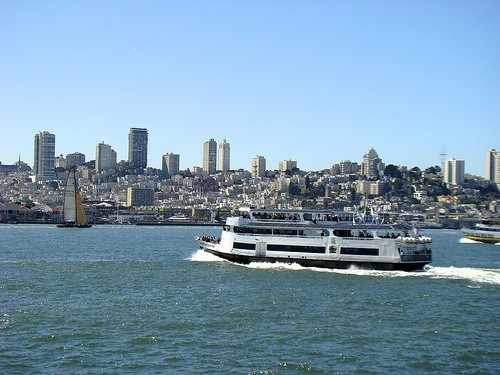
[55,166,92,228]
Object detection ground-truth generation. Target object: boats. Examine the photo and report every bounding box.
[407,215,444,229]
[461,217,500,244]
[197,205,434,271]
[166,213,195,222]
